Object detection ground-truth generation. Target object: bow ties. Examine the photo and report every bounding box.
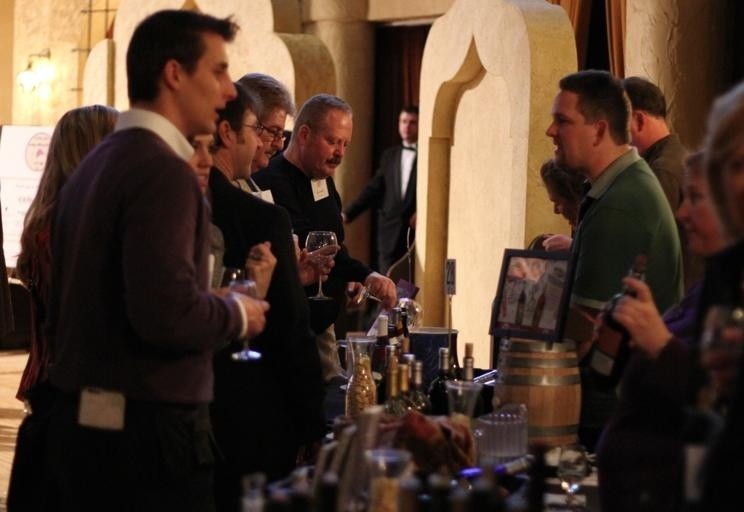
[402,145,416,152]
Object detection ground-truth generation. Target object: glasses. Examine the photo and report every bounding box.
[259,123,288,142]
[230,122,263,136]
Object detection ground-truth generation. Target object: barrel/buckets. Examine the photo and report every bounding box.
[494,330,586,449]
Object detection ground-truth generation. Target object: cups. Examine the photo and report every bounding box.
[446,381,480,414]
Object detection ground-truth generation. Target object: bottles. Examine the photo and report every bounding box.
[379,344,423,397]
[587,255,646,391]
[427,348,450,395]
[463,343,474,378]
[333,336,378,415]
[235,413,544,511]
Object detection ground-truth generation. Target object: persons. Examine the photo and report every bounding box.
[6,107,118,512]
[185,75,396,511]
[546,72,684,455]
[611,84,742,512]
[30,9,271,512]
[594,148,731,510]
[540,159,593,251]
[342,104,415,282]
[619,77,694,218]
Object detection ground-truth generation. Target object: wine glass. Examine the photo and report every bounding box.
[228,270,263,361]
[305,230,337,301]
[558,446,587,508]
